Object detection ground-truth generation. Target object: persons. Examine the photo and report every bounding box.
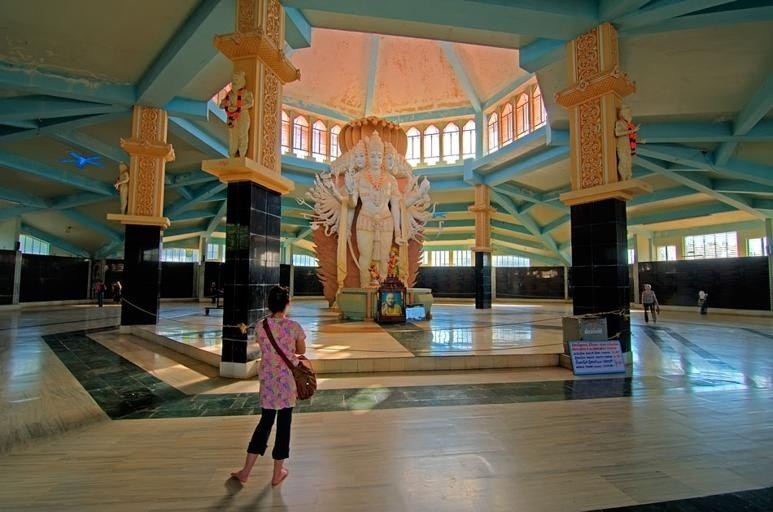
[96,280,107,307]
[615,101,645,182]
[231,285,306,486]
[383,293,402,316]
[698,288,708,315]
[218,70,254,159]
[112,281,122,304]
[303,130,438,289]
[115,161,129,215]
[642,284,660,322]
[211,283,217,304]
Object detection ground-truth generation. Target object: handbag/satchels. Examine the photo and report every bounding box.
[656,306,659,314]
[294,355,316,399]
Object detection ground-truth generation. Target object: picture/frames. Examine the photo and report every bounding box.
[379,285,406,325]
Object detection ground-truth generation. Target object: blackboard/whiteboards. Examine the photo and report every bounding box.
[567,340,626,376]
